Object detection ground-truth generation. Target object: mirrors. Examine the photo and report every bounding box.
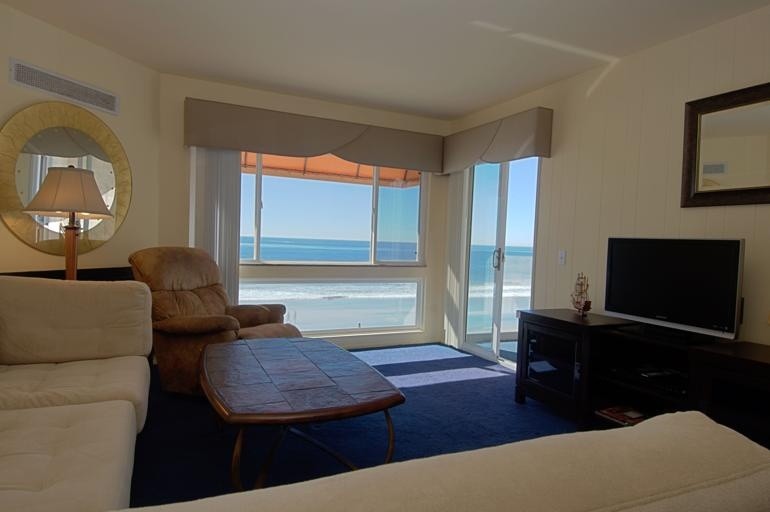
[680,81,770,208]
[0,101,132,256]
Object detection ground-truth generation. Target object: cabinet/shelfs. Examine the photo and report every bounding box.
[514,308,770,450]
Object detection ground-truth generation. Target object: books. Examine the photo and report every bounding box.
[594,406,648,427]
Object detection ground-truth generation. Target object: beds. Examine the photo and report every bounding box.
[119,409,770,512]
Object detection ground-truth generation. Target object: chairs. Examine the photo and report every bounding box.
[128,246,301,395]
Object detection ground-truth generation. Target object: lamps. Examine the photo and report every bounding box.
[21,165,113,280]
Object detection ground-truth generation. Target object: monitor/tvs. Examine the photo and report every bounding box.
[601,234,746,347]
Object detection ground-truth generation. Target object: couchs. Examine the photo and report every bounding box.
[1,275,153,512]
